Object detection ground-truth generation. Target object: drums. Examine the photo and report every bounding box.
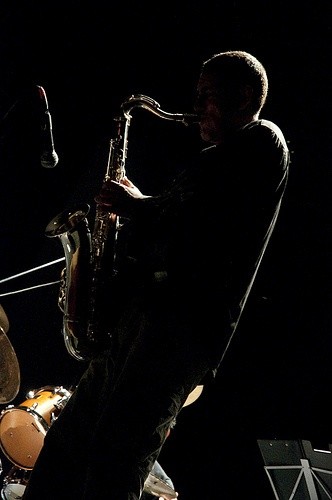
[0,476,30,500]
[0,385,75,471]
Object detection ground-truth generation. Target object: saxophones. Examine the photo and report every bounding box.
[45,90,200,360]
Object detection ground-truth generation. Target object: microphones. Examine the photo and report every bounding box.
[36,84,60,168]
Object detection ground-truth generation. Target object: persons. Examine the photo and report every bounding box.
[22,49,292,500]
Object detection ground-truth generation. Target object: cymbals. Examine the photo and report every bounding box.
[0,304,10,334]
[183,386,205,407]
[0,328,21,405]
